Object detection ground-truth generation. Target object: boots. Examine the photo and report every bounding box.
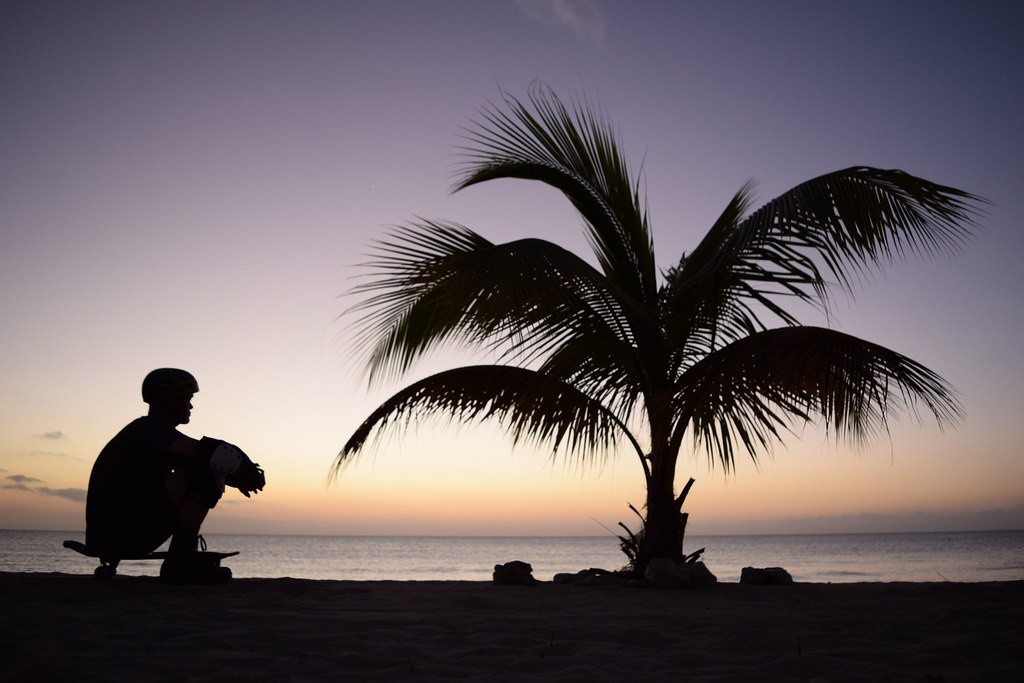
[160,528,232,585]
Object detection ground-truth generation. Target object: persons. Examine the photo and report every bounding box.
[84,368,265,577]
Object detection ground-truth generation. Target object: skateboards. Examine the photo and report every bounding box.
[61,538,241,582]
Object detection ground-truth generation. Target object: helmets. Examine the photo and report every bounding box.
[142,368,198,403]
